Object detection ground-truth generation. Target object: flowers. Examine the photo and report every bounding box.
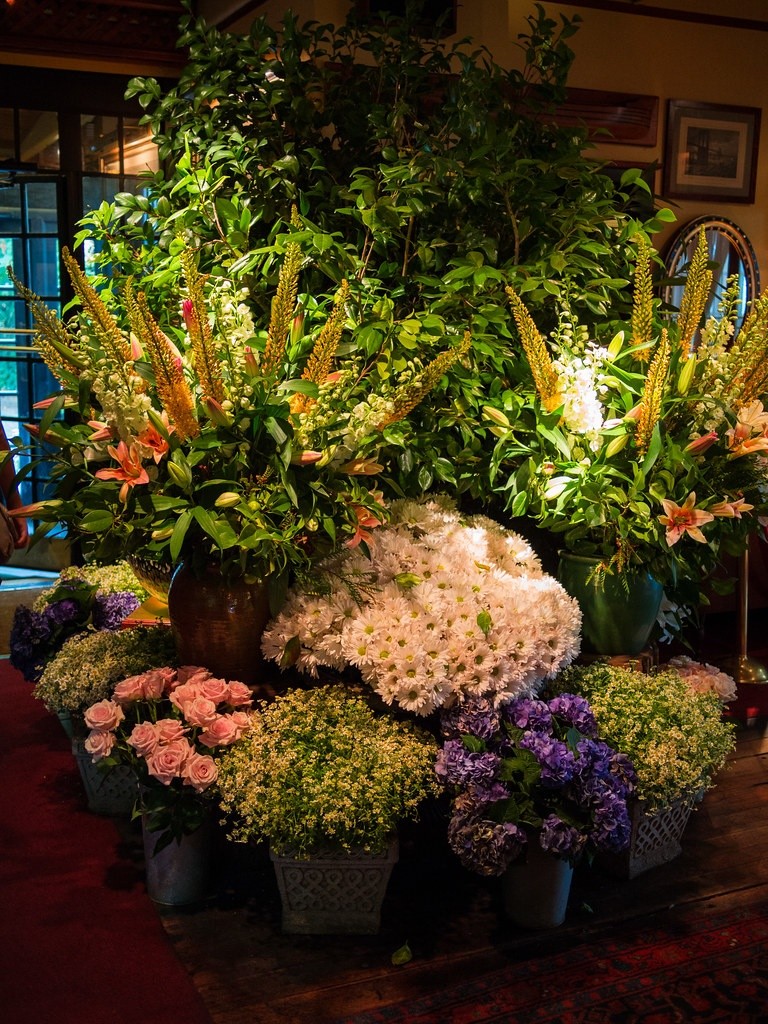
[1,222,768,877]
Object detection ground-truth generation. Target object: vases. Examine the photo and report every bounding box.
[168,558,271,686]
[557,550,664,657]
[57,712,72,739]
[137,778,212,906]
[501,856,573,927]
[128,553,170,605]
[270,825,398,935]
[612,795,694,879]
[72,737,139,816]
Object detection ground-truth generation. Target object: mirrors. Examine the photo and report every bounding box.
[658,214,758,357]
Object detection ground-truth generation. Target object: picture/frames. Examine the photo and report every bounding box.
[662,98,762,205]
